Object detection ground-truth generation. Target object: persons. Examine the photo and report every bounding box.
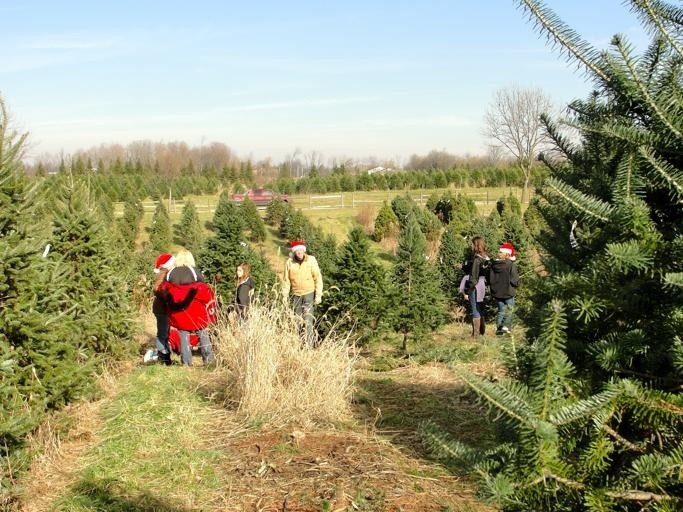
[282,241,323,347]
[229,263,254,318]
[154,248,214,367]
[465,236,520,337]
[154,253,177,365]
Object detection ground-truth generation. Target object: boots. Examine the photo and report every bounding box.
[158,351,172,365]
[471,315,485,339]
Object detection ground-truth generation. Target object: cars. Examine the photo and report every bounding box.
[232,189,289,203]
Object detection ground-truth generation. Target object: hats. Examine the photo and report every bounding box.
[173,250,195,267]
[289,242,306,258]
[499,243,516,261]
[154,254,176,273]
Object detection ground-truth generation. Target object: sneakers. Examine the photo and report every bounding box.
[495,326,510,336]
